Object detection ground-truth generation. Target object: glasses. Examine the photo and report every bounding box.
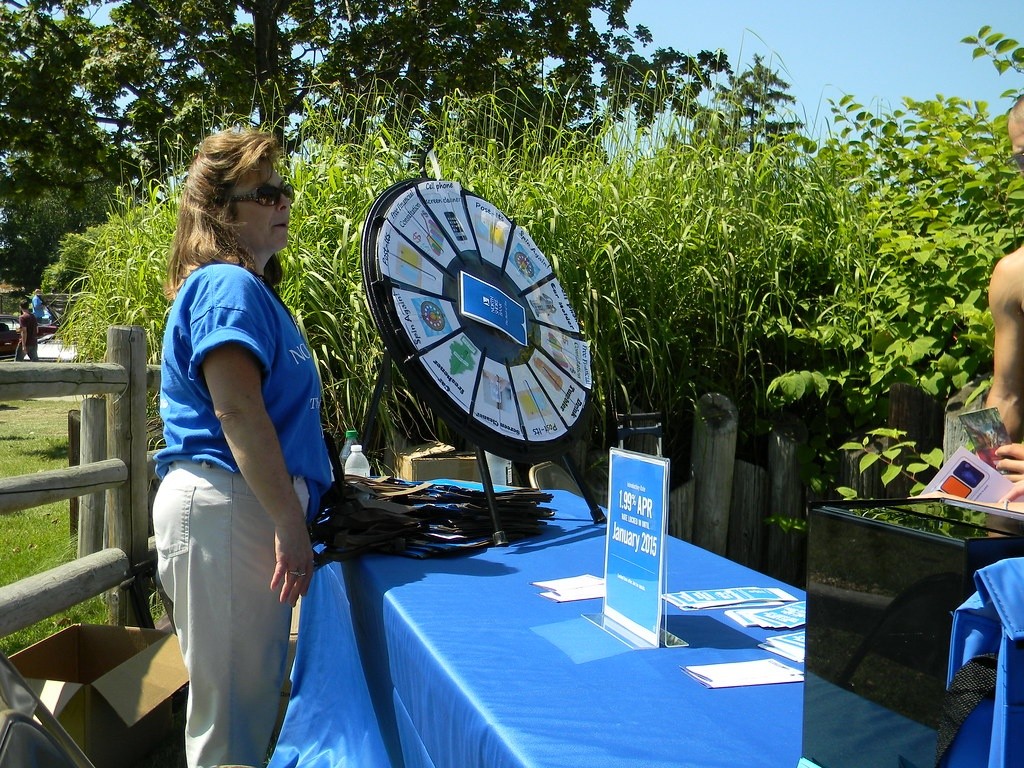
[222,183,295,207]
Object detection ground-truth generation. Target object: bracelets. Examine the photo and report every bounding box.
[1003,499,1010,510]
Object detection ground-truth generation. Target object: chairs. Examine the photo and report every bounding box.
[0,650,97,768]
[529,461,584,502]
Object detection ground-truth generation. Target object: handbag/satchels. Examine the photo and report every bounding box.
[931,556,1024,768]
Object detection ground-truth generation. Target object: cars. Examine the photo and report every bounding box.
[0,315,59,360]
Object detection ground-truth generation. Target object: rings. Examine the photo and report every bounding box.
[288,571,306,577]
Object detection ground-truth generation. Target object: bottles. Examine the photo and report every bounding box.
[339,430,358,475]
[345,445,370,500]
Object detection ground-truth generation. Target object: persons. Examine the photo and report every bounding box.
[907,96,1024,514]
[14,299,38,362]
[151,127,333,768]
[32,288,61,325]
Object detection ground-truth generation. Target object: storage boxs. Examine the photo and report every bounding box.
[3,623,190,768]
[383,441,483,485]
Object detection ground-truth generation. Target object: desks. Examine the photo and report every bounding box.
[266,477,808,768]
